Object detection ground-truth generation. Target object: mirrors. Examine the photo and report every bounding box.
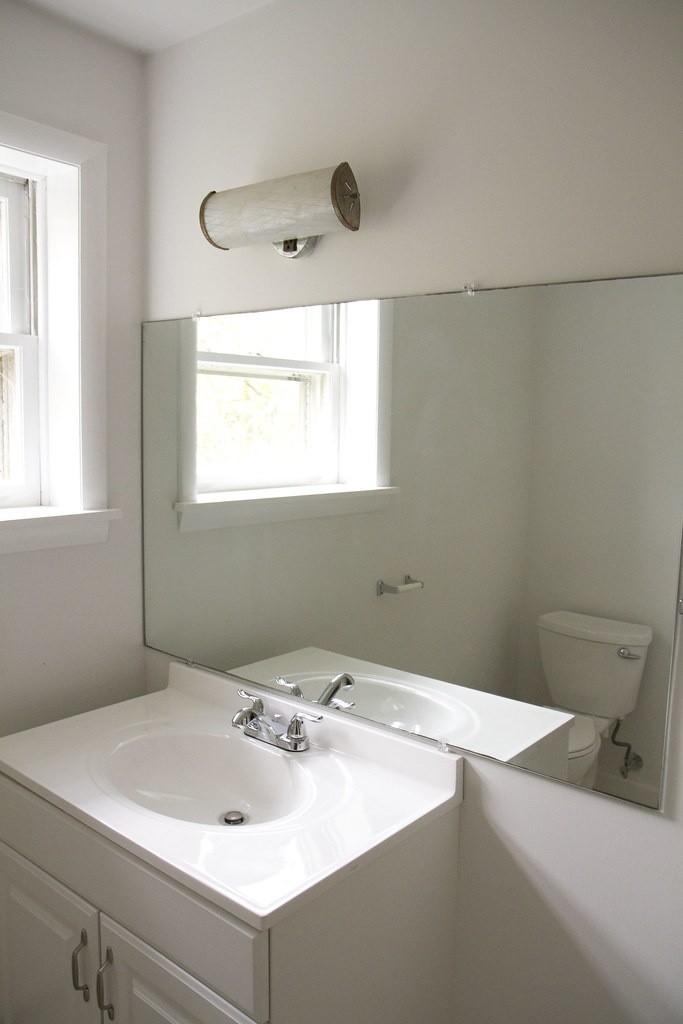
[142,274,682,824]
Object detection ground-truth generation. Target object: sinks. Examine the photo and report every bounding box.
[266,669,463,739]
[106,730,318,828]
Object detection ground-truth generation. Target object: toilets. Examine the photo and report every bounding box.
[538,610,654,790]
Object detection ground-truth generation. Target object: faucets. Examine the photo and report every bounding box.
[316,671,356,706]
[231,706,282,745]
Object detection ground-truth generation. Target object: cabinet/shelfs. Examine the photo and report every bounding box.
[0,770,462,1024]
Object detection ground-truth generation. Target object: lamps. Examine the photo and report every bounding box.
[198,160,362,260]
